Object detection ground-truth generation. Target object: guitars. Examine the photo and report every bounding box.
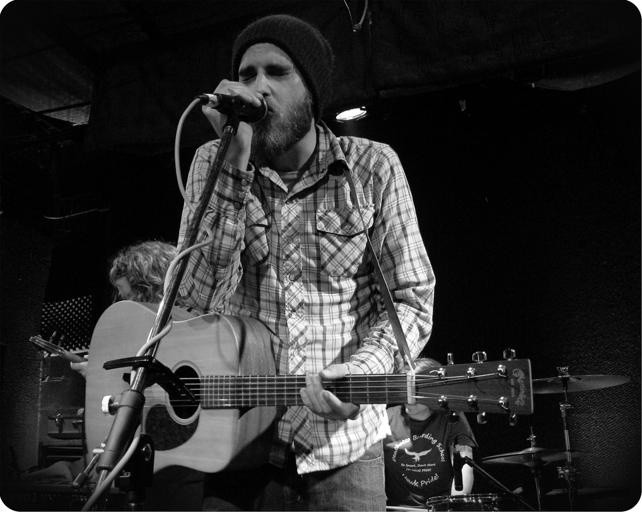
[28,330,87,363]
[85,299,536,488]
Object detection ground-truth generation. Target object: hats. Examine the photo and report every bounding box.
[231,15,332,125]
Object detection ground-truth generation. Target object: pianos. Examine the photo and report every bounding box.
[1,472,129,511]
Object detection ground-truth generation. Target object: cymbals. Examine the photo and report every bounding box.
[532,374,632,395]
[479,446,595,470]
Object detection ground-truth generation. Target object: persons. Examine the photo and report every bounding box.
[385,357,478,506]
[69,240,178,380]
[172,14,437,512]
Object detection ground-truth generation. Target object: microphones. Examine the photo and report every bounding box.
[453,438,463,491]
[198,94,268,123]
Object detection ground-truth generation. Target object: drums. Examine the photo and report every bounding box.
[425,492,500,512]
